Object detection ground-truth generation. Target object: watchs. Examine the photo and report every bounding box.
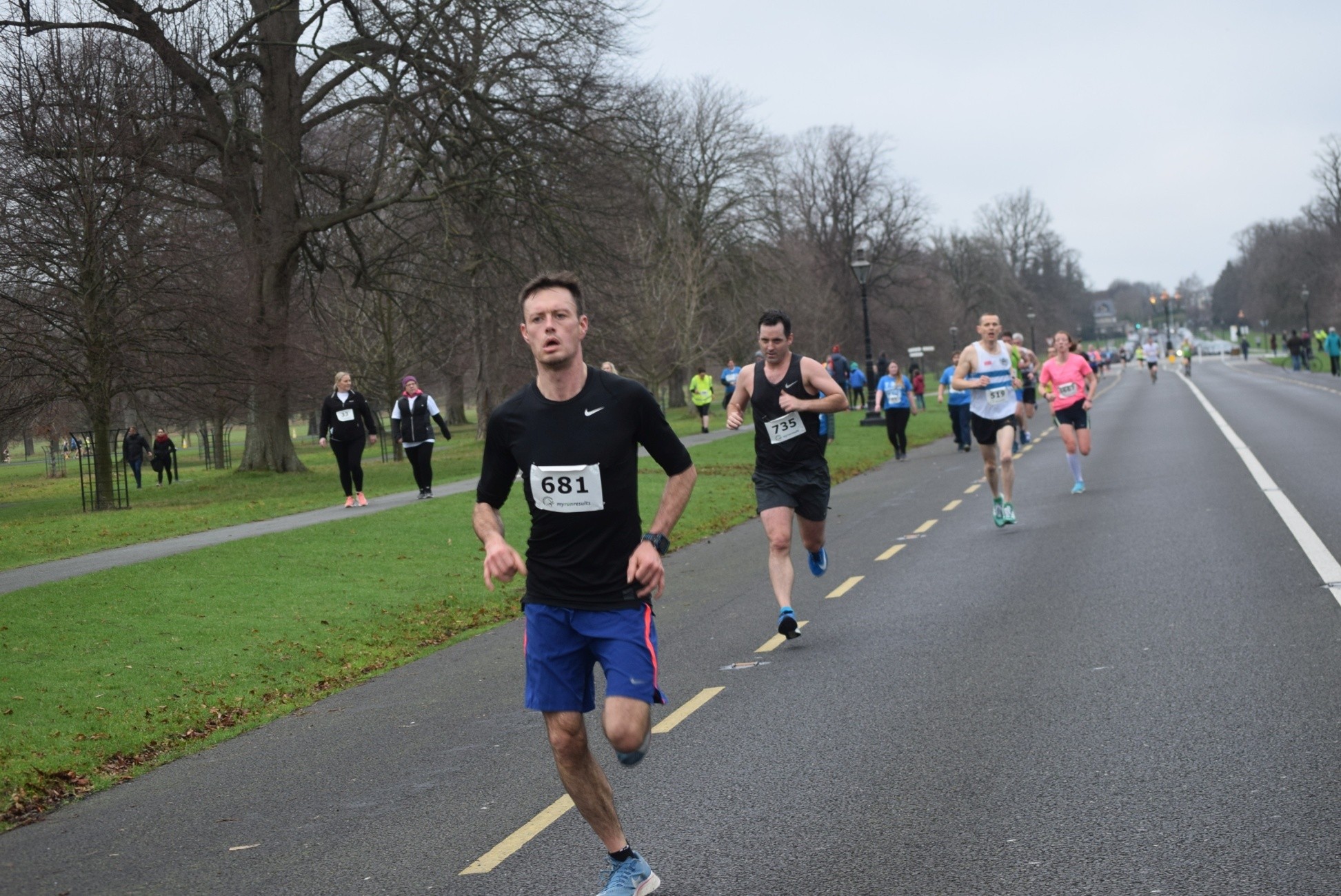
[644,532,670,556]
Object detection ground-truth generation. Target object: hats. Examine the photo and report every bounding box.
[851,362,858,368]
[833,345,839,353]
[402,375,417,387]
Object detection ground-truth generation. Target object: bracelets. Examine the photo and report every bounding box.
[1043,393,1046,398]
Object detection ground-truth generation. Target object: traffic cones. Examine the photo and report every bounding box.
[183,439,188,448]
[291,429,297,438]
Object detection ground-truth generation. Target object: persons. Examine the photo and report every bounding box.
[1143,339,1160,384]
[391,377,452,498]
[817,343,869,456]
[153,428,176,487]
[601,362,619,376]
[62,436,91,460]
[319,372,377,507]
[874,361,918,462]
[1181,339,1192,376]
[123,426,153,489]
[1270,333,1278,356]
[689,350,764,434]
[1281,326,1341,377]
[725,310,849,638]
[1094,344,1145,373]
[1241,337,1250,360]
[872,353,927,412]
[3,446,11,463]
[938,312,1101,528]
[471,272,697,896]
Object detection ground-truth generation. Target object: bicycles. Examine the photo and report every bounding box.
[1281,350,1323,374]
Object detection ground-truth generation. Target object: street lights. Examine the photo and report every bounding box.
[1300,284,1312,345]
[1025,310,1037,356]
[1238,310,1245,333]
[845,246,886,425]
[1150,289,1182,350]
[949,326,958,350]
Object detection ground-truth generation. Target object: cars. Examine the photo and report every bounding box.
[1201,346,1220,356]
[1120,328,1158,362]
[1097,347,1122,364]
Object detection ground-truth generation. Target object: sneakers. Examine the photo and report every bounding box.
[1003,502,1016,525]
[596,850,661,896]
[992,493,1006,528]
[778,611,802,640]
[356,492,367,506]
[418,489,425,499]
[615,726,651,768]
[808,547,828,577]
[423,487,433,499]
[344,495,354,507]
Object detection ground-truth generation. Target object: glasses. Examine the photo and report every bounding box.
[406,383,416,387]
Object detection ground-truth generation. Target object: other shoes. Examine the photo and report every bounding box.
[964,444,971,452]
[1012,441,1019,454]
[702,427,704,432]
[704,428,708,432]
[1019,429,1030,444]
[900,453,906,461]
[895,446,901,459]
[1071,481,1086,495]
